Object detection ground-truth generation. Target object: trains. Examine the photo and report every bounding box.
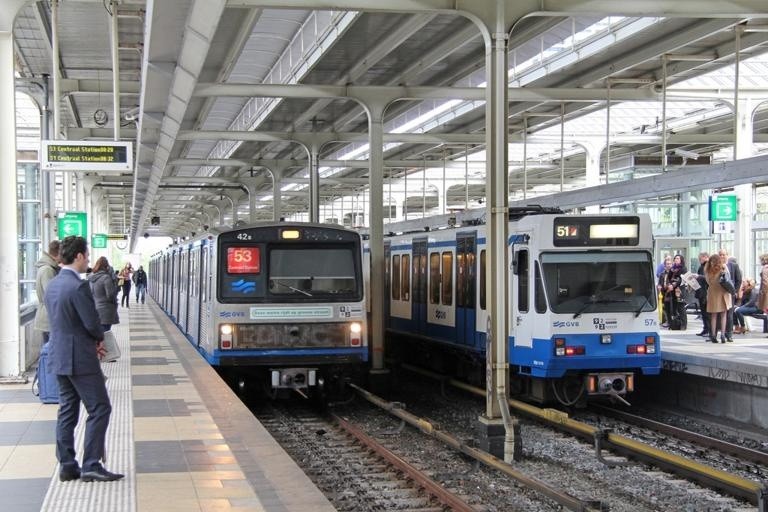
[351,204,663,409]
[147,220,369,408]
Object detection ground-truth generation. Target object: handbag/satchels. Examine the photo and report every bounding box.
[719,271,736,295]
[32,341,64,404]
[102,331,122,362]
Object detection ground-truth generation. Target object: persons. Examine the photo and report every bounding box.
[41,235,124,482]
[32,240,62,345]
[87,255,148,362]
[656,248,768,343]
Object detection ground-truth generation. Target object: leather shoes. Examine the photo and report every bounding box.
[59,466,81,482]
[81,465,125,482]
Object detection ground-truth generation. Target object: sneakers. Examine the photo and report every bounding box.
[696,326,746,344]
